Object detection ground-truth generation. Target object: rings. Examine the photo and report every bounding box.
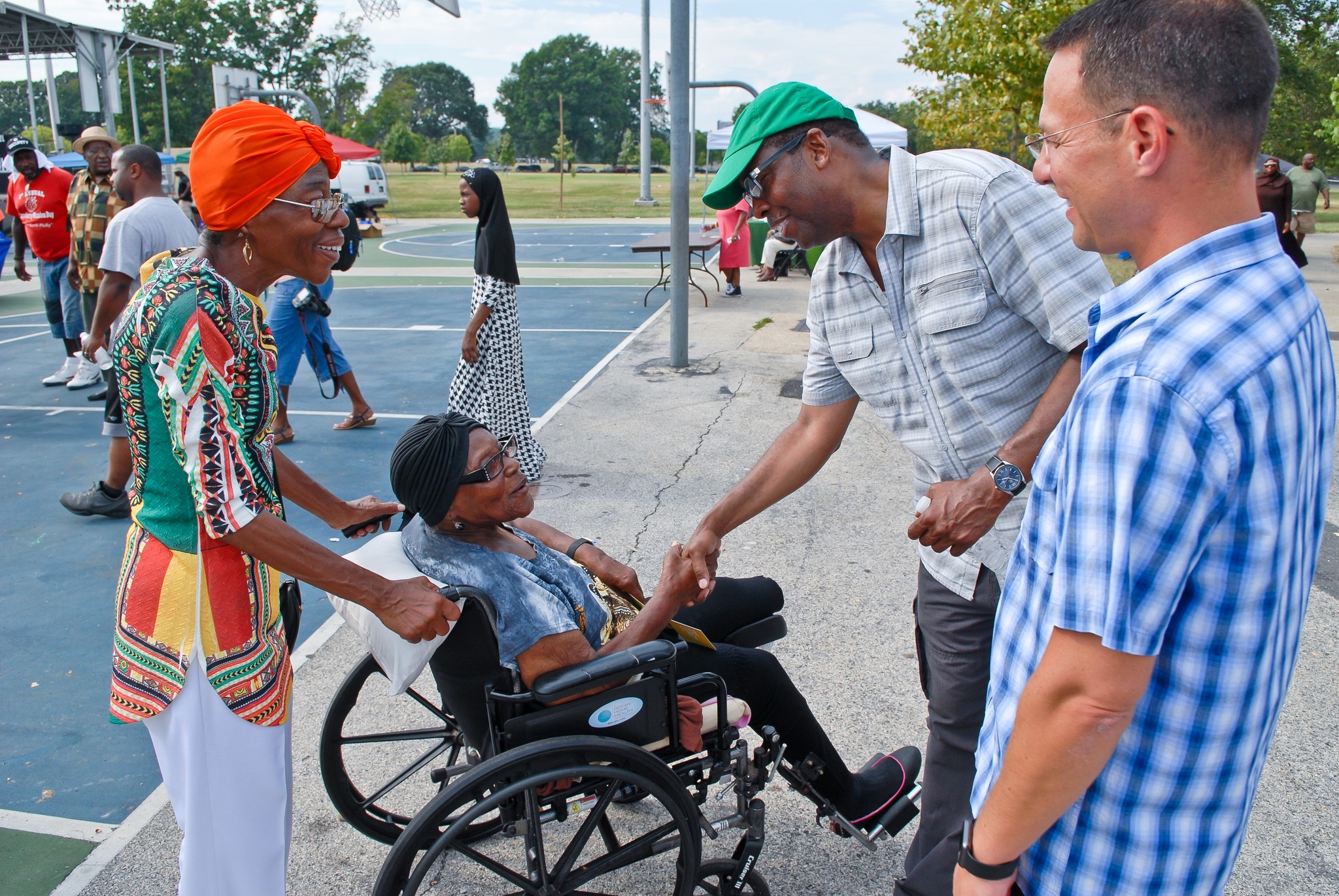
[84,352,88,354]
[767,235,769,237]
[734,238,736,239]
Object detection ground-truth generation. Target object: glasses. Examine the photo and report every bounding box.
[1024,110,1174,160]
[267,193,345,223]
[742,131,831,209]
[84,147,111,157]
[1264,162,1276,167]
[458,433,518,485]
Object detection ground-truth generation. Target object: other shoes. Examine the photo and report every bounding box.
[88,386,108,401]
[830,746,922,838]
[756,266,764,273]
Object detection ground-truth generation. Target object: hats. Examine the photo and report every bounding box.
[6,136,36,156]
[73,126,120,155]
[701,82,859,210]
[174,166,183,172]
[389,411,492,527]
[189,100,341,232]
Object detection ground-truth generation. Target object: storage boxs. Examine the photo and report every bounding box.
[357,222,385,237]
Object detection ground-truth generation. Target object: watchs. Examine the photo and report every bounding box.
[985,456,1026,496]
[957,818,1021,880]
[567,537,594,559]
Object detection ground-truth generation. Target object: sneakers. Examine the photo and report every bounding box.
[66,359,101,390]
[727,283,733,292]
[723,286,742,297]
[42,357,83,386]
[59,482,131,518]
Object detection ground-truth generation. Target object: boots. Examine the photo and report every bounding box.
[757,267,775,281]
[757,267,768,277]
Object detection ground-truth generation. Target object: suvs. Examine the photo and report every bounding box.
[576,166,596,173]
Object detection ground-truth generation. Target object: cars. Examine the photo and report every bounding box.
[455,162,567,173]
[599,164,668,174]
[695,165,716,173]
[410,163,440,172]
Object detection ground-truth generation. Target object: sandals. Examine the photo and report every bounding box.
[273,424,294,445]
[333,407,377,429]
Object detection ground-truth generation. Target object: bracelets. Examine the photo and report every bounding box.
[14,257,23,260]
[714,223,717,227]
[710,225,712,230]
[772,230,775,233]
[1325,199,1329,202]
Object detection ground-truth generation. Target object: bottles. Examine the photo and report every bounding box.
[700,220,707,238]
[915,498,1016,577]
[79,332,113,370]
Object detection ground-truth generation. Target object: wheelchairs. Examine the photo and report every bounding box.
[317,506,922,896]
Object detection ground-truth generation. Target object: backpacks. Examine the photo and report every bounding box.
[331,200,363,271]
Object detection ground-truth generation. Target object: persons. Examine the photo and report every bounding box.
[449,168,547,481]
[679,83,1114,896]
[107,100,461,896]
[1255,153,1330,245]
[952,0,1339,896]
[391,411,921,827]
[702,198,799,297]
[4,126,377,517]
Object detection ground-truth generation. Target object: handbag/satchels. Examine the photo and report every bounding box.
[279,580,302,657]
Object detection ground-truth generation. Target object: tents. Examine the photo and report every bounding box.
[326,133,398,222]
[173,151,191,200]
[46,151,177,168]
[704,106,908,232]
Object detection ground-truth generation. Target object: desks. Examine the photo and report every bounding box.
[631,231,721,308]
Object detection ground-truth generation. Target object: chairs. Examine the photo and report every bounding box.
[774,243,812,281]
